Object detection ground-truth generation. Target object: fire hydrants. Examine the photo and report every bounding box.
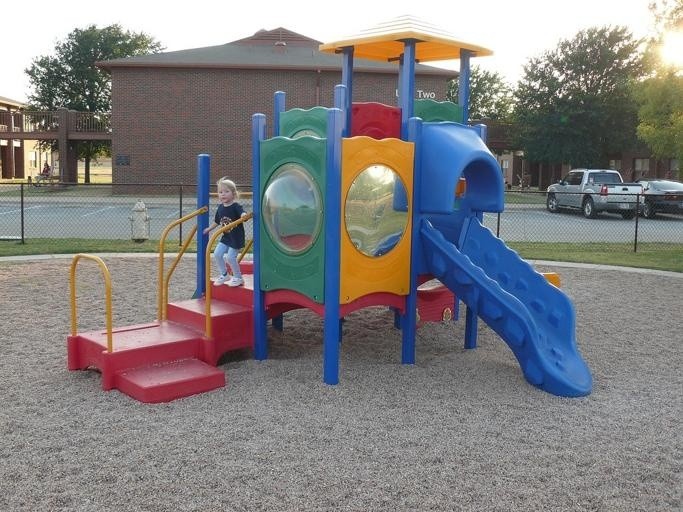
[127,199,151,243]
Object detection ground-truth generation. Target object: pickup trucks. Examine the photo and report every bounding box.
[545,167,646,220]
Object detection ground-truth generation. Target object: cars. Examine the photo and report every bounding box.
[634,178,683,220]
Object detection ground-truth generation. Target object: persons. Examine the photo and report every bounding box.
[34,163,51,187]
[202,175,249,287]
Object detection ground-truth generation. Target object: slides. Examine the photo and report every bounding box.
[421,216,594,398]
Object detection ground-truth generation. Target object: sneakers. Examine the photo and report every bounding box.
[213,273,231,287]
[223,276,245,287]
